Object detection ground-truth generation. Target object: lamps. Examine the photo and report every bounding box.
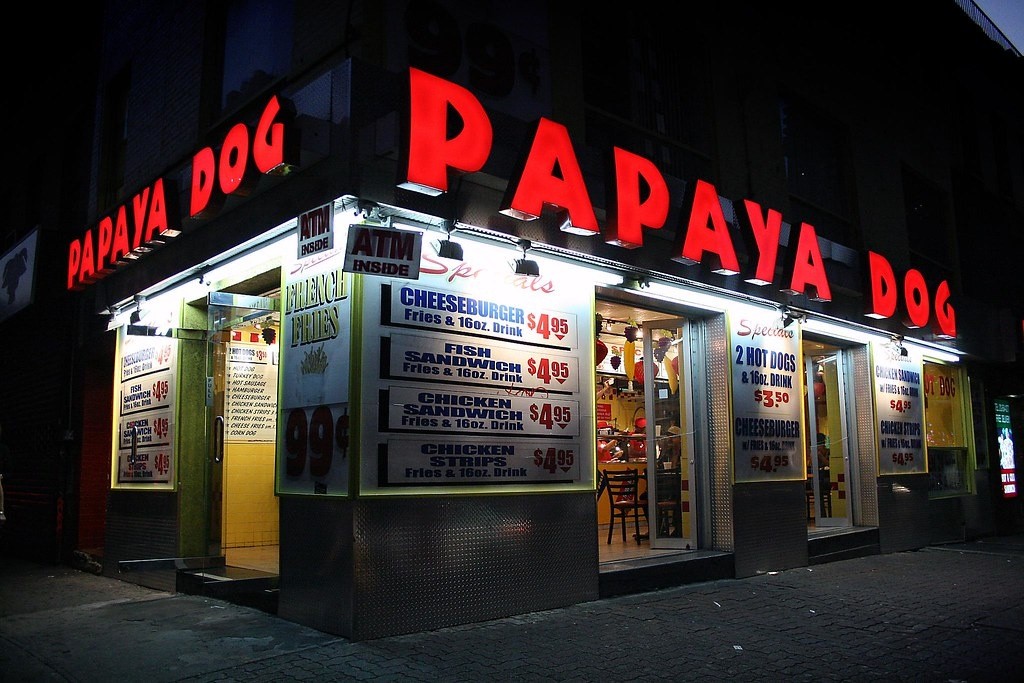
[129,295,150,324]
[778,304,794,328]
[104,307,124,331]
[892,336,909,356]
[508,239,539,276]
[430,219,464,261]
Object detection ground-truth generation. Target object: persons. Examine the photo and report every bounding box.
[998,428,1014,468]
[632,425,683,541]
[808,433,832,517]
[628,418,647,459]
[596,420,623,462]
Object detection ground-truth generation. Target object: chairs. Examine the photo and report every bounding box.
[603,468,681,545]
[805,462,832,522]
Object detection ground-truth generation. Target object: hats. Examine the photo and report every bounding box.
[668,426,680,434]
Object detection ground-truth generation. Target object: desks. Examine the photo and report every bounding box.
[615,472,682,536]
